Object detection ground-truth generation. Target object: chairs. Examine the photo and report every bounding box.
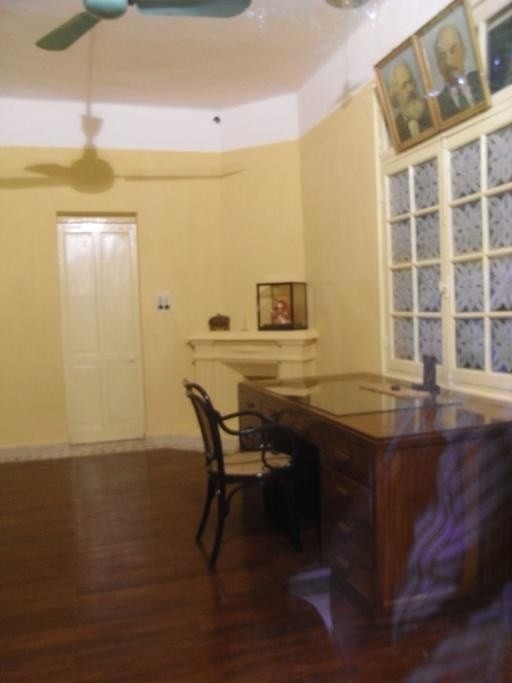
[181,374,305,572]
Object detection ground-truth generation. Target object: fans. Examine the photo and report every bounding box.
[35,0,254,52]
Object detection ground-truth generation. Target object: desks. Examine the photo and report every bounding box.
[236,370,512,634]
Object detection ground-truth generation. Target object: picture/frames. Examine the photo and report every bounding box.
[416,0,494,135]
[370,30,439,157]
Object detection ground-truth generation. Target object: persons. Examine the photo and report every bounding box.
[387,61,433,140]
[432,22,482,120]
